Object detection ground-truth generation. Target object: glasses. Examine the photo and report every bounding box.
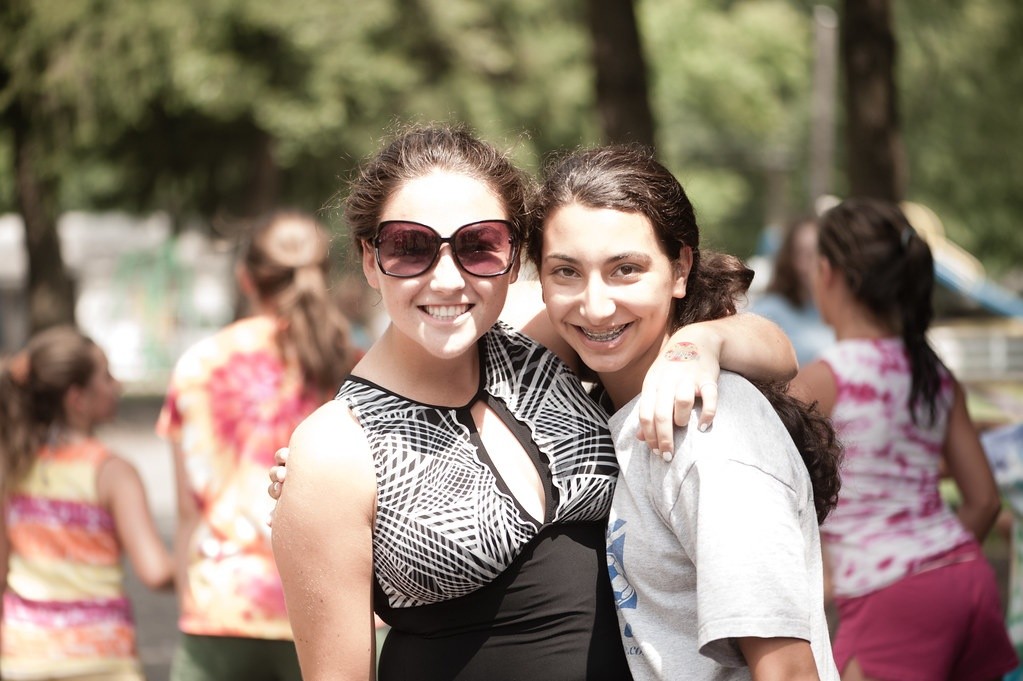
[371,218,522,278]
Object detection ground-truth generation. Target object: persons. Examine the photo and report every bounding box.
[271,124,802,681]
[265,144,841,681]
[0,323,175,680]
[154,208,388,680]
[756,194,1021,679]
